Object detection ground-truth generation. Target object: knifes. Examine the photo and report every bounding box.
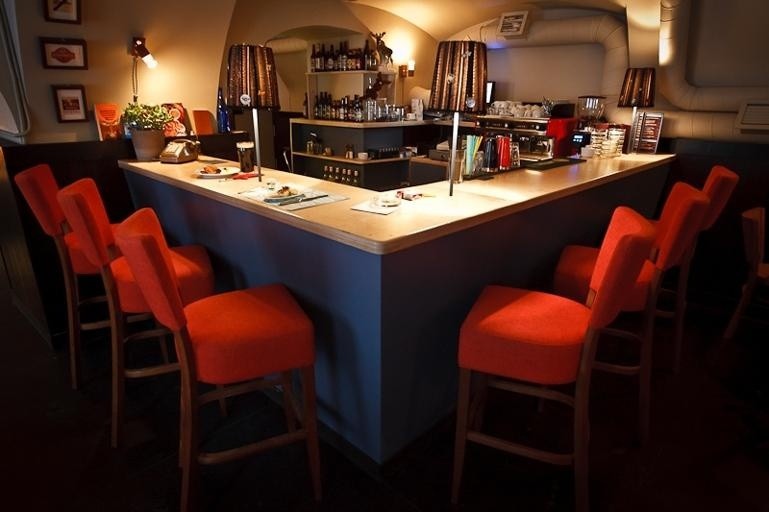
[278,194,328,205]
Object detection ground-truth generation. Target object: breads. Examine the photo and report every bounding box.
[383,200,401,207]
[204,165,217,174]
[271,186,298,198]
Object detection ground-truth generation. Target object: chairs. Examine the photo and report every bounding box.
[109,204,328,511]
[54,174,216,452]
[550,177,712,448]
[644,163,741,380]
[722,202,769,347]
[11,162,125,392]
[448,203,664,511]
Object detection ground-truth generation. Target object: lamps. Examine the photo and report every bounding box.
[424,39,488,197]
[617,67,656,153]
[224,43,281,182]
[399,60,415,104]
[124,35,160,107]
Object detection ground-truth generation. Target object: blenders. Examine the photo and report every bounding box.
[570,94,608,154]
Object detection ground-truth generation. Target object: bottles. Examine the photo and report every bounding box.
[482,134,510,173]
[302,92,309,118]
[322,166,360,186]
[217,87,231,134]
[345,143,354,159]
[312,89,363,121]
[309,39,377,72]
[365,99,405,121]
[377,147,399,158]
[417,98,424,121]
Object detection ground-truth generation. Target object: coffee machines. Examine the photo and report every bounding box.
[474,114,553,167]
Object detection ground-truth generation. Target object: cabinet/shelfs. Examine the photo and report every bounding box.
[289,117,431,166]
[306,68,399,119]
[474,113,576,161]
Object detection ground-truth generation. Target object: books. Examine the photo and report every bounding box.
[92,103,213,142]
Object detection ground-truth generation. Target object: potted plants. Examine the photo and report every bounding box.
[120,102,176,163]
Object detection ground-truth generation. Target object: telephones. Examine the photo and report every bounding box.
[159,139,201,163]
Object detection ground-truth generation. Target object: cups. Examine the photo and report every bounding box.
[306,140,315,154]
[447,149,465,185]
[510,142,521,169]
[490,98,544,118]
[235,140,256,173]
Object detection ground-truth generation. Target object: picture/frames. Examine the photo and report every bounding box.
[43,0,82,26]
[50,82,91,124]
[37,35,89,70]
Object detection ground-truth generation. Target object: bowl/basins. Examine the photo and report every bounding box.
[357,152,368,160]
[581,147,595,158]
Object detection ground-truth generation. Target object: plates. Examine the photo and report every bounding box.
[193,167,241,178]
[263,192,303,200]
